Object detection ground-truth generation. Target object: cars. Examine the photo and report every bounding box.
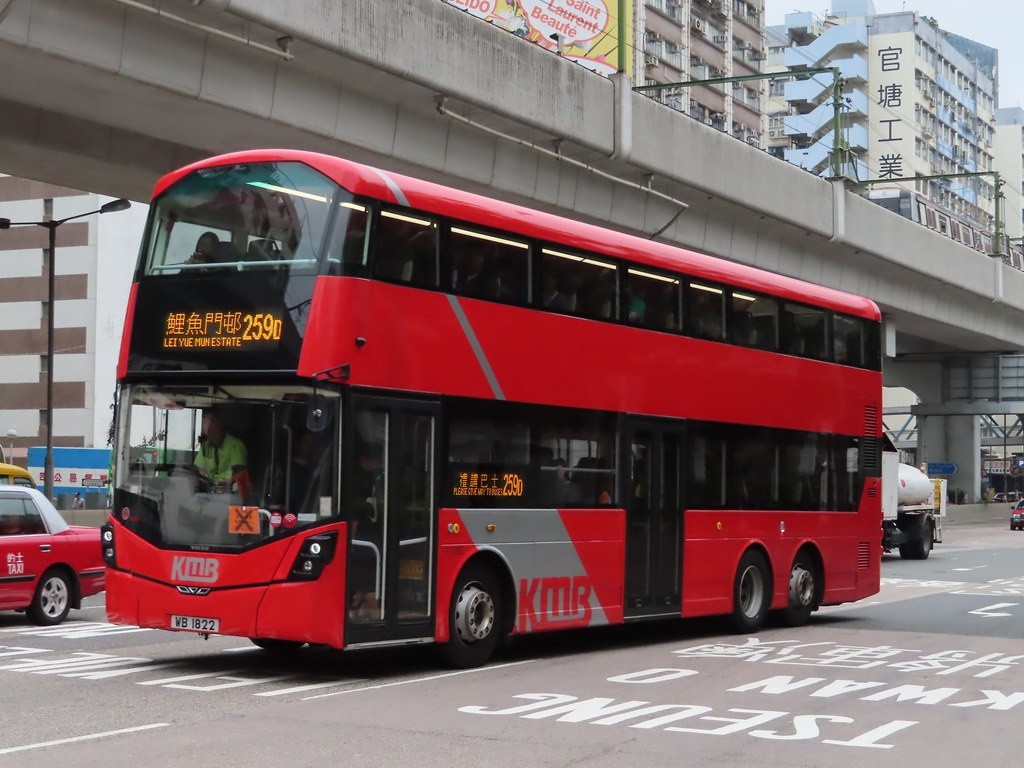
[992,492,1017,502]
[1009,498,1023,530]
[0,461,105,625]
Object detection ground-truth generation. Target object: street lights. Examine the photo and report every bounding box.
[1,199,133,504]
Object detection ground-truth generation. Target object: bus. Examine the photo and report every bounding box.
[101,149,884,674]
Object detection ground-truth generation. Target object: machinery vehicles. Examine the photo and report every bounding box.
[882,450,948,560]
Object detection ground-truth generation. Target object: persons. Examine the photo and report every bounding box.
[178,231,224,275]
[190,415,245,495]
[71,491,84,509]
